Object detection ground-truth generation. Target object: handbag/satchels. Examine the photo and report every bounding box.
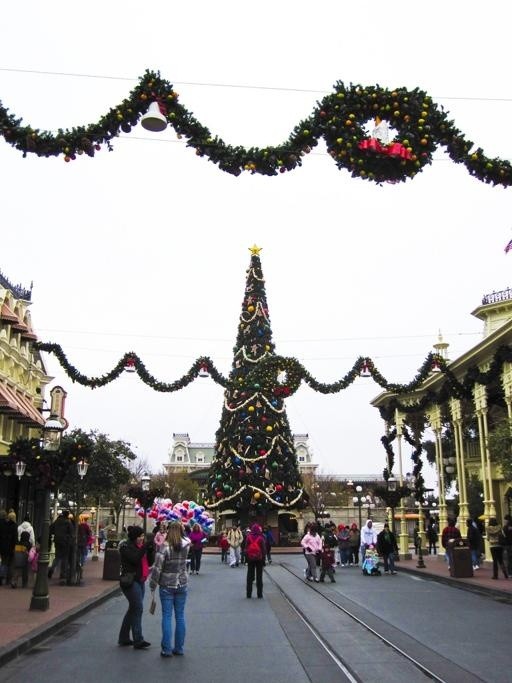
[499,534,507,546]
[118,571,136,587]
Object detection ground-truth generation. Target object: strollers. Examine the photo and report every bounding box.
[362,545,381,575]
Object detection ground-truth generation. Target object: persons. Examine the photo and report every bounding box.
[300,515,512,582]
[0,508,107,589]
[149,522,191,658]
[117,526,151,648]
[152,522,209,574]
[220,522,277,567]
[244,523,264,600]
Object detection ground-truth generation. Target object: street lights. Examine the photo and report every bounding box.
[351,480,364,534]
[10,453,28,524]
[317,500,329,527]
[89,503,96,532]
[135,466,151,552]
[66,451,91,586]
[362,490,377,522]
[29,407,63,612]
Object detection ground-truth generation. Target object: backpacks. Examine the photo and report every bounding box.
[245,535,264,565]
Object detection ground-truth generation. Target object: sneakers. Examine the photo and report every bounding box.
[188,558,240,578]
[133,640,151,649]
[117,638,132,645]
[306,561,398,585]
[1,571,83,589]
[473,565,512,579]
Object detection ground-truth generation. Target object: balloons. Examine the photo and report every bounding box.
[135,496,215,547]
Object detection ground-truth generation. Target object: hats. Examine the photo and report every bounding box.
[251,522,262,533]
[127,525,146,539]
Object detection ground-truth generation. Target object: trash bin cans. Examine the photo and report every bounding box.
[447,538,473,578]
[103,540,120,580]
